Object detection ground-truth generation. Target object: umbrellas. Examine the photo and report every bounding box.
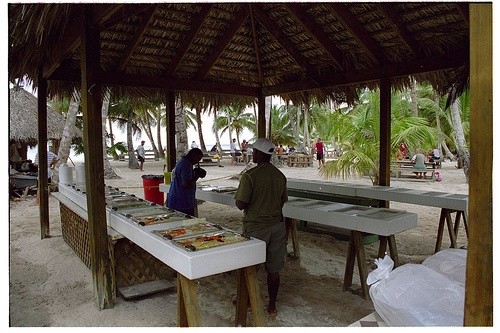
[10,86,84,141]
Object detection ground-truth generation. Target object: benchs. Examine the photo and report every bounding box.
[395,168,437,180]
[429,158,444,169]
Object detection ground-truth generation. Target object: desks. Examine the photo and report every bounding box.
[389,160,436,178]
[49,176,469,327]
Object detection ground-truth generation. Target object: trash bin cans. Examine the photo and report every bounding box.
[142,175,164,206]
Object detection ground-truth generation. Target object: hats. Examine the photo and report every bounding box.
[248,138,276,154]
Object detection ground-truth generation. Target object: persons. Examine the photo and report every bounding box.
[230,138,252,162]
[275,144,295,155]
[400,141,407,156]
[314,138,326,169]
[236,138,290,317]
[34,151,60,203]
[136,140,145,170]
[411,149,426,179]
[211,145,218,152]
[167,148,206,218]
[428,147,440,159]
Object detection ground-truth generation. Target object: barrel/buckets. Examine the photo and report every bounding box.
[75,163,86,182]
[59,166,73,183]
